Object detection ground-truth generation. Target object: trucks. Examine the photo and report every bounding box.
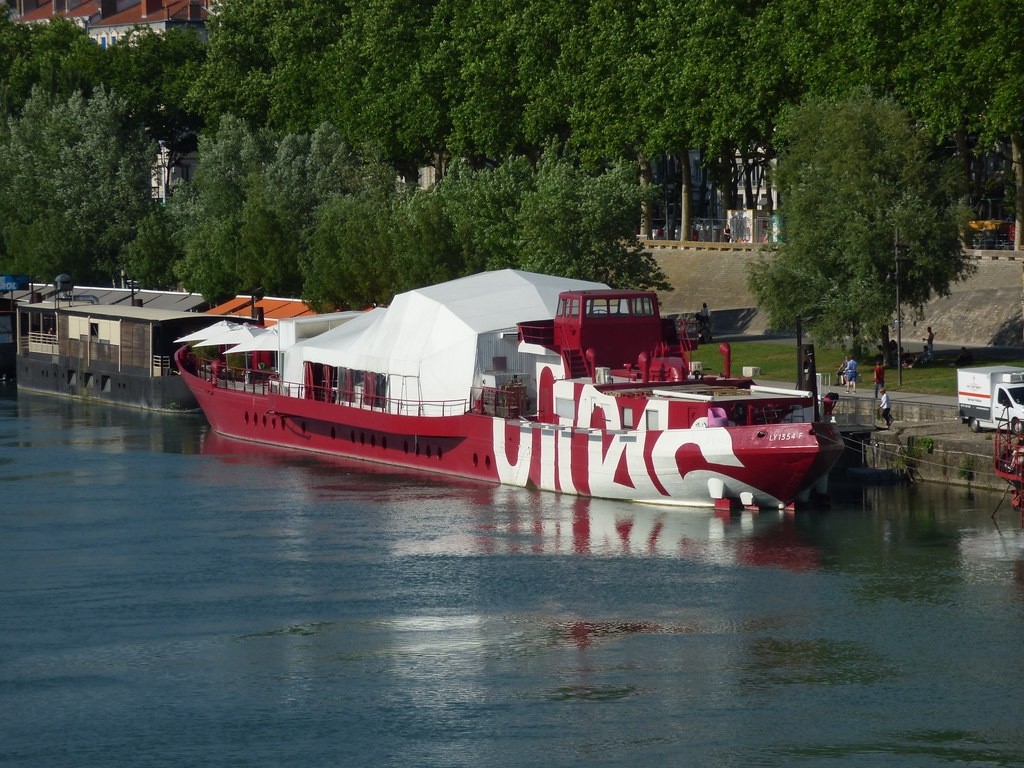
[957,365,1024,434]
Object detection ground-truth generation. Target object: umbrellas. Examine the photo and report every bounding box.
[172,319,240,371]
[222,328,279,372]
[193,322,270,369]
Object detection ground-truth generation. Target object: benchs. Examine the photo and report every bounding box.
[841,374,862,385]
[742,367,761,377]
[689,362,703,371]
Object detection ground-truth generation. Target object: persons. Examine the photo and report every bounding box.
[982,231,996,248]
[835,356,850,386]
[876,389,894,426]
[701,303,711,329]
[723,225,732,242]
[879,326,934,369]
[845,354,857,394]
[875,361,884,398]
[48,326,53,334]
[949,347,973,366]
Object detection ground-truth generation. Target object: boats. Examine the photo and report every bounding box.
[15,274,264,413]
[173,269,845,510]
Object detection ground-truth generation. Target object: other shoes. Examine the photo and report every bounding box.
[845,391,849,394]
[852,391,856,394]
